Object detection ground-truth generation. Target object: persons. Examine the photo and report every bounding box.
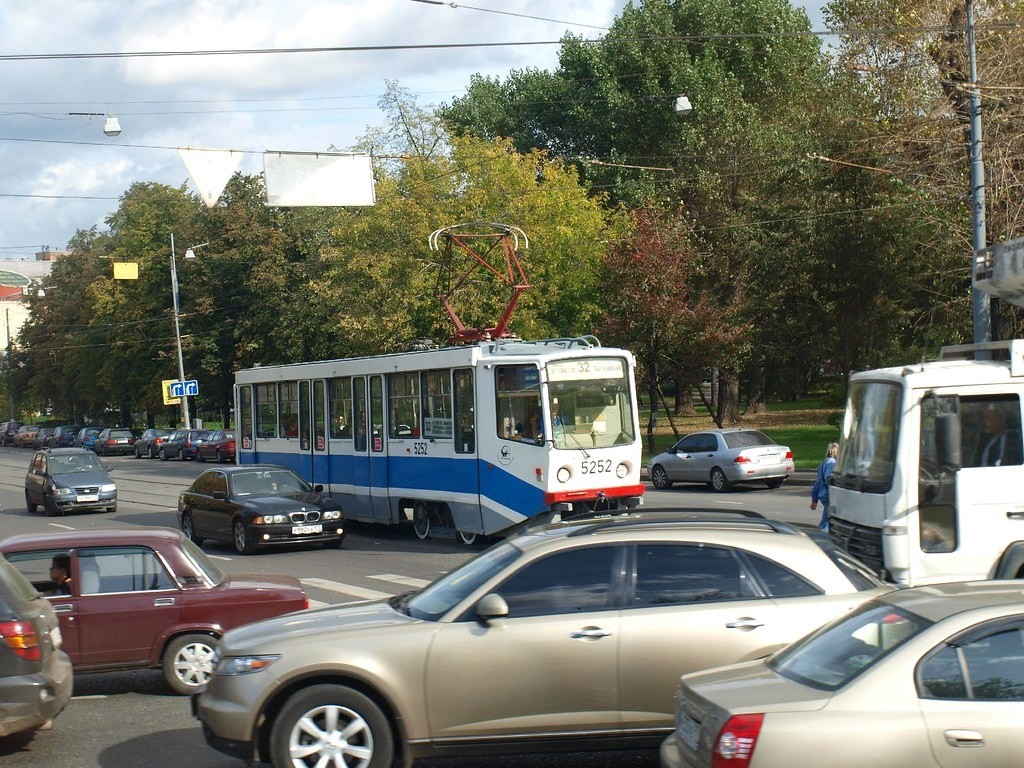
[40,552,72,594]
[274,407,298,438]
[358,410,365,434]
[810,443,840,534]
[537,395,570,435]
[333,414,350,436]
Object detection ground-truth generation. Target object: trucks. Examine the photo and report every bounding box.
[824,237,1024,593]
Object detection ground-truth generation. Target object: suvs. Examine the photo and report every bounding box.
[0,422,25,447]
[191,505,897,768]
[0,551,76,743]
[25,445,118,516]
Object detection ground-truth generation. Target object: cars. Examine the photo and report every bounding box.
[657,578,1024,768]
[195,429,235,464]
[645,428,795,494]
[134,427,178,459]
[176,464,346,552]
[12,425,45,449]
[0,525,311,699]
[32,427,56,450]
[48,425,80,449]
[72,427,104,453]
[158,428,206,462]
[92,428,136,456]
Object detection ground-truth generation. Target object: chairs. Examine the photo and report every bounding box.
[79,554,100,594]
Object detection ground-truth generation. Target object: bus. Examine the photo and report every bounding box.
[233,221,645,548]
